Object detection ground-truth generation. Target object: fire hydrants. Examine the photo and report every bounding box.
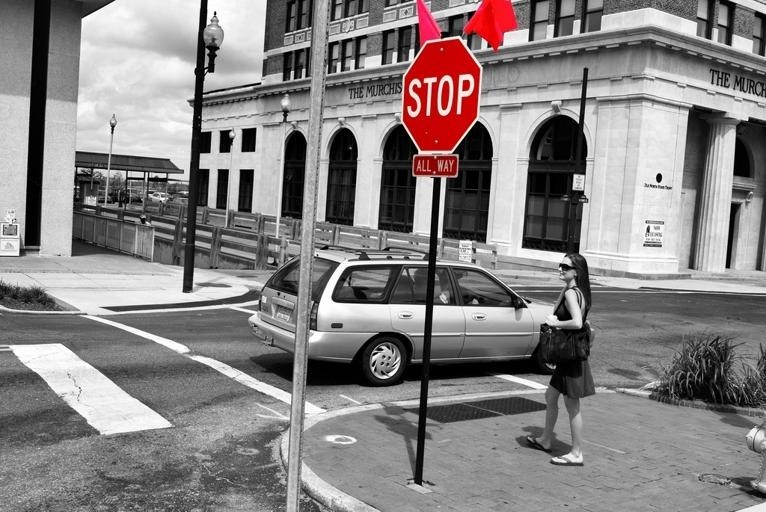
[740,421,766,496]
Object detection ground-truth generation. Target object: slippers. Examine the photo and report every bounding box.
[551,455,584,466]
[526,436,552,454]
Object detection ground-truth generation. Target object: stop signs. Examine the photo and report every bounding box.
[400,36,483,154]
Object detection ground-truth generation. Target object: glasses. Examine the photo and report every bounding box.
[559,263,575,271]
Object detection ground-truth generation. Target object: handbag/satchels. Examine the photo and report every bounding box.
[541,322,590,363]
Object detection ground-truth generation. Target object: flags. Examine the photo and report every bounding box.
[416,0,442,50]
[462,3,520,53]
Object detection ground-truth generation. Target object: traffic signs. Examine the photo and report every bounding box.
[412,155,458,178]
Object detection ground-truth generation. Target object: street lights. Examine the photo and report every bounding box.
[171,1,229,294]
[224,128,237,229]
[270,94,293,268]
[98,113,120,216]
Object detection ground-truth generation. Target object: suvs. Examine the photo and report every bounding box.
[243,239,593,389]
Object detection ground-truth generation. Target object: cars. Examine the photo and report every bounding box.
[74,186,189,206]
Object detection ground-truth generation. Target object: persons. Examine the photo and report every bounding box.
[527,252,595,464]
